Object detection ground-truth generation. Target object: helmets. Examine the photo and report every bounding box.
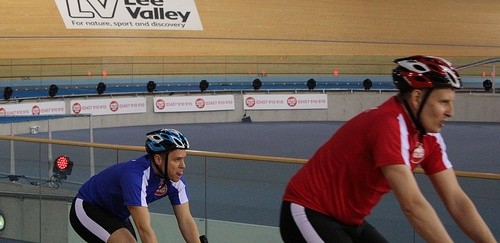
[392,55,462,91]
[145,129,190,154]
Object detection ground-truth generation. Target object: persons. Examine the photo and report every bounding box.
[279,54,496,243]
[70,129,201,242]
